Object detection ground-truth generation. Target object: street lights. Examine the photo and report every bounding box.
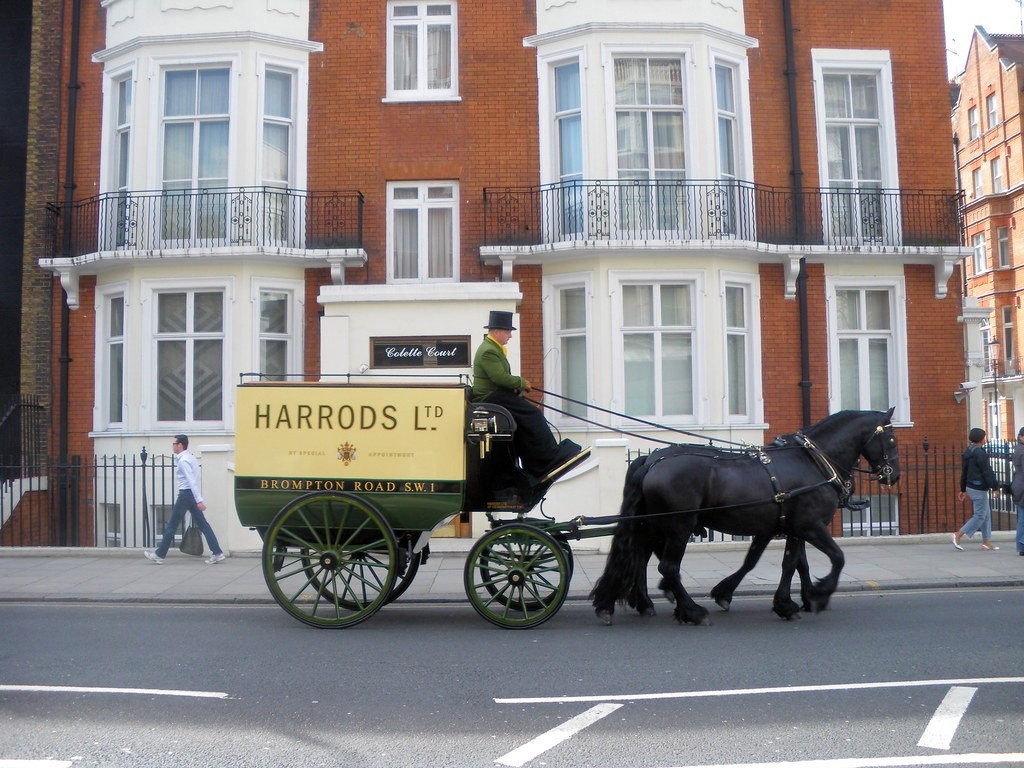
[989,335,1000,440]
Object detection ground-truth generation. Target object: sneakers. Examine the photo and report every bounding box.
[204,552,226,564]
[144,550,166,565]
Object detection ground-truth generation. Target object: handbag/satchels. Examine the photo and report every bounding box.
[178,513,204,556]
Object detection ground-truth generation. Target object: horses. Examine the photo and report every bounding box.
[589,405,901,627]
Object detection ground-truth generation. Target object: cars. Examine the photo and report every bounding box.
[982,441,1018,494]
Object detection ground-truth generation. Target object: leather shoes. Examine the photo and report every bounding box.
[982,544,1000,550]
[951,533,964,551]
[1020,552,1024,556]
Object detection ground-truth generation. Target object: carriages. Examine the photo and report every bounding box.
[234,373,901,629]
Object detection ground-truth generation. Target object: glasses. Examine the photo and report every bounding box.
[173,443,179,445]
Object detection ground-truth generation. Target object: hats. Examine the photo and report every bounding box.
[482,311,517,330]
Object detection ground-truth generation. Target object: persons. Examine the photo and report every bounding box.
[952,428,1000,551]
[1011,426,1024,556]
[144,435,226,564]
[472,311,562,470]
